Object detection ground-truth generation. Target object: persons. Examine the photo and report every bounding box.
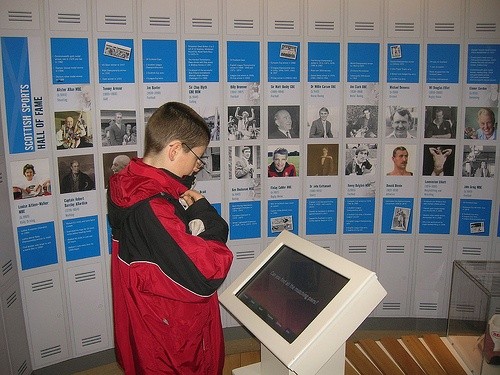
[385,146,413,176]
[346,145,375,175]
[429,146,452,176]
[465,109,497,140]
[60,160,95,193]
[347,109,377,137]
[267,147,297,177]
[269,110,298,139]
[57,117,93,150]
[229,106,260,140]
[424,109,456,139]
[309,107,335,138]
[234,147,255,179]
[106,101,234,375]
[314,145,335,176]
[386,107,416,138]
[110,155,132,174]
[19,164,46,199]
[105,111,136,145]
[475,160,493,177]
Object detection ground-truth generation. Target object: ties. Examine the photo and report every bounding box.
[287,132,290,137]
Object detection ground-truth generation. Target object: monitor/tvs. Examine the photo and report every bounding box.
[218,230,388,375]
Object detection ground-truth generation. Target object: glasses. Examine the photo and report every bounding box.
[170,142,207,170]
